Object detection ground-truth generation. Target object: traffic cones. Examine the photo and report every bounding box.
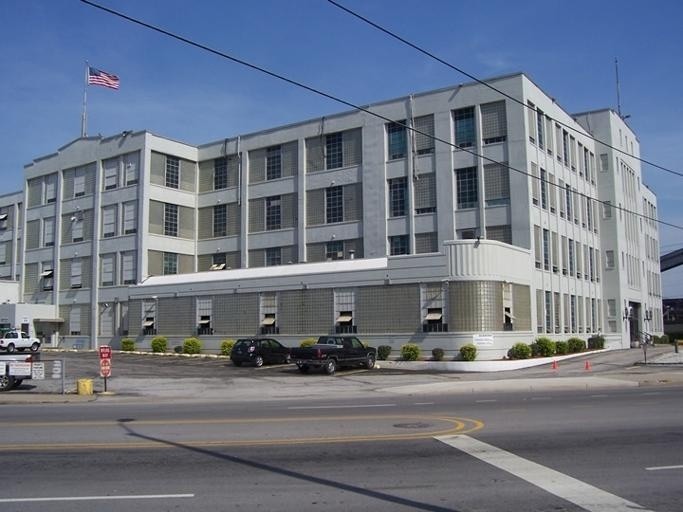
[551,360,559,369]
[583,359,592,370]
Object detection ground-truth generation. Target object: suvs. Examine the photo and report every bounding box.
[229,337,291,367]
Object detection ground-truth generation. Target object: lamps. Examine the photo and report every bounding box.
[622,307,654,322]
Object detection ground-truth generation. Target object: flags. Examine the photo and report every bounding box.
[89,66,120,91]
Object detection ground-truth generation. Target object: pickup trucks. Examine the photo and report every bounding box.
[0,330,41,352]
[287,331,377,374]
[0,350,41,391]
